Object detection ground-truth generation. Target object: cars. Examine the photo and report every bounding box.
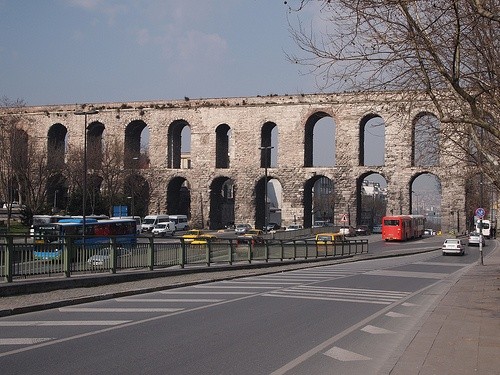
[180,228,230,244]
[235,224,252,234]
[467,232,485,246]
[373,225,382,233]
[285,225,302,231]
[340,226,355,237]
[87,246,132,267]
[442,239,466,256]
[267,223,279,230]
[311,220,326,228]
[238,229,264,237]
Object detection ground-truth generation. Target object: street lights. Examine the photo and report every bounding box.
[73,110,99,246]
[258,145,274,234]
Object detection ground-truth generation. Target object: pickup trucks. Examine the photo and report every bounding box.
[354,224,373,235]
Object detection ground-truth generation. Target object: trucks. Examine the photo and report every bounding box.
[141,215,169,232]
[152,222,175,237]
[169,215,188,230]
[110,216,141,234]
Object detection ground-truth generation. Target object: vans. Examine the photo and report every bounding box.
[315,232,349,255]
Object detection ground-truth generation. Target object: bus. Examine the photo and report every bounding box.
[381,214,426,242]
[32,214,109,226]
[475,219,491,239]
[32,219,138,264]
